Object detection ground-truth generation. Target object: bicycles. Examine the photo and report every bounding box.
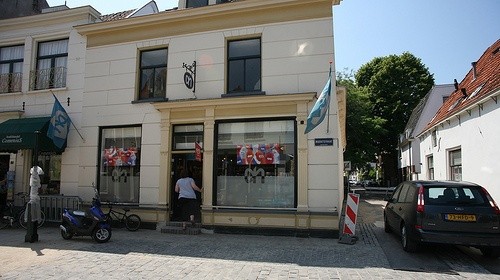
[1,191,44,228]
[102,200,141,232]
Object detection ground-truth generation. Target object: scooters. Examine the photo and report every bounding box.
[60,199,111,243]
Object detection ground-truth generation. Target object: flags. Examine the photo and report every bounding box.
[303,62,331,135]
[47,90,70,149]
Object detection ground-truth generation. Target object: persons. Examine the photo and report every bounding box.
[175,168,204,230]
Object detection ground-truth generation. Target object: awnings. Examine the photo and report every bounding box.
[0,117,67,154]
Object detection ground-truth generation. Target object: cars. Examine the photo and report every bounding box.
[383,179,500,257]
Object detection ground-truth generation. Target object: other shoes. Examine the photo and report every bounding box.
[183,226,187,230]
[190,221,195,226]
